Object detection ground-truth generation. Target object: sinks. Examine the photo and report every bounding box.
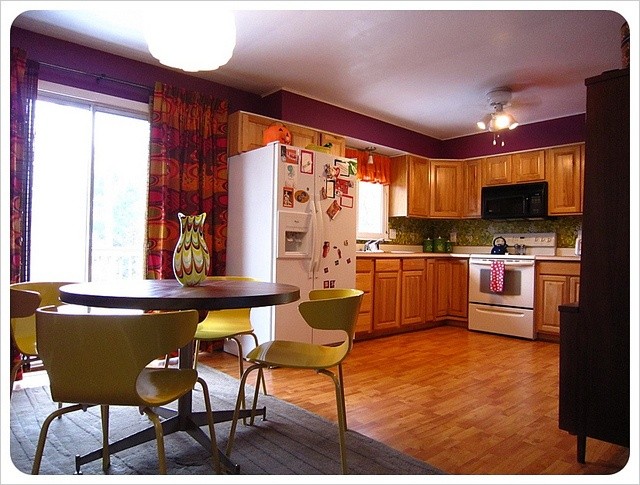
[357,251,414,255]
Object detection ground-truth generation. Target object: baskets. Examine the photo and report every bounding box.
[306,143,332,155]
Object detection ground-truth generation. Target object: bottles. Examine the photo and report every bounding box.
[433,234,446,252]
[446,240,453,253]
[422,237,433,252]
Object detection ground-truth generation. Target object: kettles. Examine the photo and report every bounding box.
[490,236,508,254]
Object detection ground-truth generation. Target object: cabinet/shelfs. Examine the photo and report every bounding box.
[535,260,581,344]
[431,159,464,218]
[355,258,374,342]
[556,66,629,465]
[545,142,585,216]
[485,150,546,186]
[449,257,469,328]
[322,131,347,158]
[464,155,483,220]
[427,257,449,328]
[389,154,430,218]
[401,257,426,334]
[227,110,321,158]
[374,258,401,339]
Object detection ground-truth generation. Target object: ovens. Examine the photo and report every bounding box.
[470,256,536,337]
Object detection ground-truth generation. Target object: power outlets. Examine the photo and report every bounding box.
[450,233,457,242]
[389,228,397,240]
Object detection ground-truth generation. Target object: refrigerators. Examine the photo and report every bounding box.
[222,140,358,366]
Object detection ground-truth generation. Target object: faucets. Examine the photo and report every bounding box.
[372,238,387,250]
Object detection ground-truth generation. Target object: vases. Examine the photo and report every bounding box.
[173,210,212,287]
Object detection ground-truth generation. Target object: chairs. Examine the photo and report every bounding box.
[226,287,365,475]
[10,282,76,398]
[31,304,221,475]
[165,276,267,426]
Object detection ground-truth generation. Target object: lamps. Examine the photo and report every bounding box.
[365,145,376,165]
[496,110,509,130]
[476,112,494,130]
[488,115,500,134]
[506,112,519,132]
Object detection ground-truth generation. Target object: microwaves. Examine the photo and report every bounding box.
[481,181,548,219]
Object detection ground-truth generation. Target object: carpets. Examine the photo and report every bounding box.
[10,357,444,474]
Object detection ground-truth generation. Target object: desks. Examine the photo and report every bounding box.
[58,279,301,475]
